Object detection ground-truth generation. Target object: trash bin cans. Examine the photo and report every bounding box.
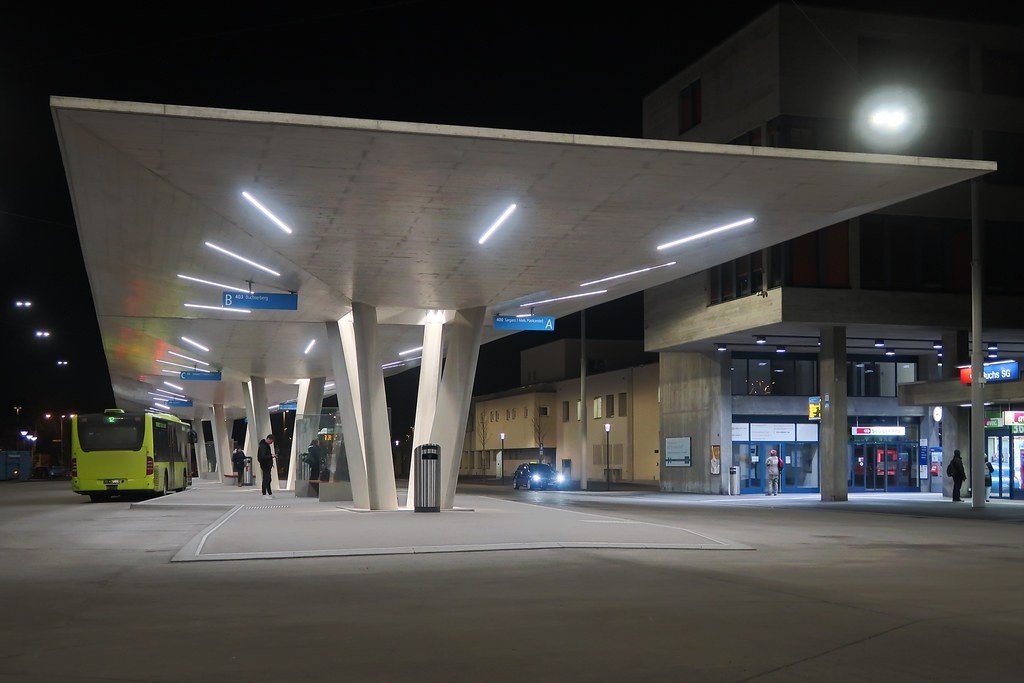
[604,469,612,481]
[244,456,252,486]
[414,444,441,513]
[730,465,741,496]
[612,469,622,482]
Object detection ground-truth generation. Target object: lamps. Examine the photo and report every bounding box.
[756,337,766,343]
[933,341,942,349]
[988,343,997,350]
[937,356,942,366]
[875,339,884,347]
[937,349,942,356]
[988,351,997,357]
[776,346,786,352]
[885,349,896,356]
[718,345,726,350]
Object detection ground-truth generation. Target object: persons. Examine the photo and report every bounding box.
[950,450,967,502]
[257,434,278,499]
[984,453,994,502]
[231,447,247,487]
[308,440,321,480]
[993,452,1003,462]
[766,450,779,496]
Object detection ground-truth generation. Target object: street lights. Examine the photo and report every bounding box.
[500,432,504,485]
[604,424,610,491]
[60,414,64,466]
[861,94,988,509]
[13,406,22,415]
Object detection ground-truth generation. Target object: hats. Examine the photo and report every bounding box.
[771,450,776,455]
[984,453,987,458]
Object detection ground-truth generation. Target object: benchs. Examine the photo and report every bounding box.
[295,480,351,502]
[224,474,256,486]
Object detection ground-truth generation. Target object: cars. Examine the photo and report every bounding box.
[513,462,564,491]
[36,467,63,480]
[989,463,1020,494]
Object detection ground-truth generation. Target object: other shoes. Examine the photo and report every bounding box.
[953,499,964,502]
[765,493,771,496]
[774,494,777,495]
[263,495,275,499]
[986,500,989,502]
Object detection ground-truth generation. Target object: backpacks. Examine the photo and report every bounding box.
[778,458,783,471]
[985,462,990,477]
[947,459,958,476]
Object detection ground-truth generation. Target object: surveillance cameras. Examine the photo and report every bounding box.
[756,291,764,296]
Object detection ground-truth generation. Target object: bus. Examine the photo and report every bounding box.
[855,449,896,476]
[72,408,197,502]
